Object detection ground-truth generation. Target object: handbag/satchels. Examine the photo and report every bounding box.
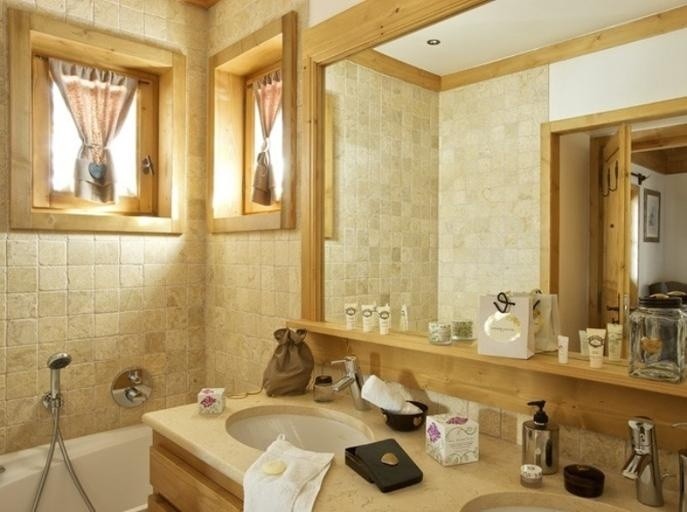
[513,290,560,353]
[477,292,536,359]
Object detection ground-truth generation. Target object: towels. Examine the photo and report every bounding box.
[361,375,423,415]
[243,440,335,512]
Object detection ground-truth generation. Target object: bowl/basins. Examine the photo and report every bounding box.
[378,400,428,431]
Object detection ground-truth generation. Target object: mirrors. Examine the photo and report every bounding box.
[296,0,687,382]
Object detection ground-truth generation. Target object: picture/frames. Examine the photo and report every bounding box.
[644,188,661,242]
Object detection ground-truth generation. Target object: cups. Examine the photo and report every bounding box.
[451,318,474,340]
[427,321,453,345]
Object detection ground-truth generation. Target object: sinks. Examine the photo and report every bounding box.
[224,404,375,457]
[458,491,631,512]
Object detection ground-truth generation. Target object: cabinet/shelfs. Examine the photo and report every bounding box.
[148,429,244,512]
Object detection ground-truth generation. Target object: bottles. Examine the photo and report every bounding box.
[627,295,686,384]
[312,375,336,402]
[645,291,686,375]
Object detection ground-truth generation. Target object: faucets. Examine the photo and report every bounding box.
[622,414,677,508]
[678,449,687,512]
[330,355,372,411]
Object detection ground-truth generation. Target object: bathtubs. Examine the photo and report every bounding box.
[0,423,154,512]
